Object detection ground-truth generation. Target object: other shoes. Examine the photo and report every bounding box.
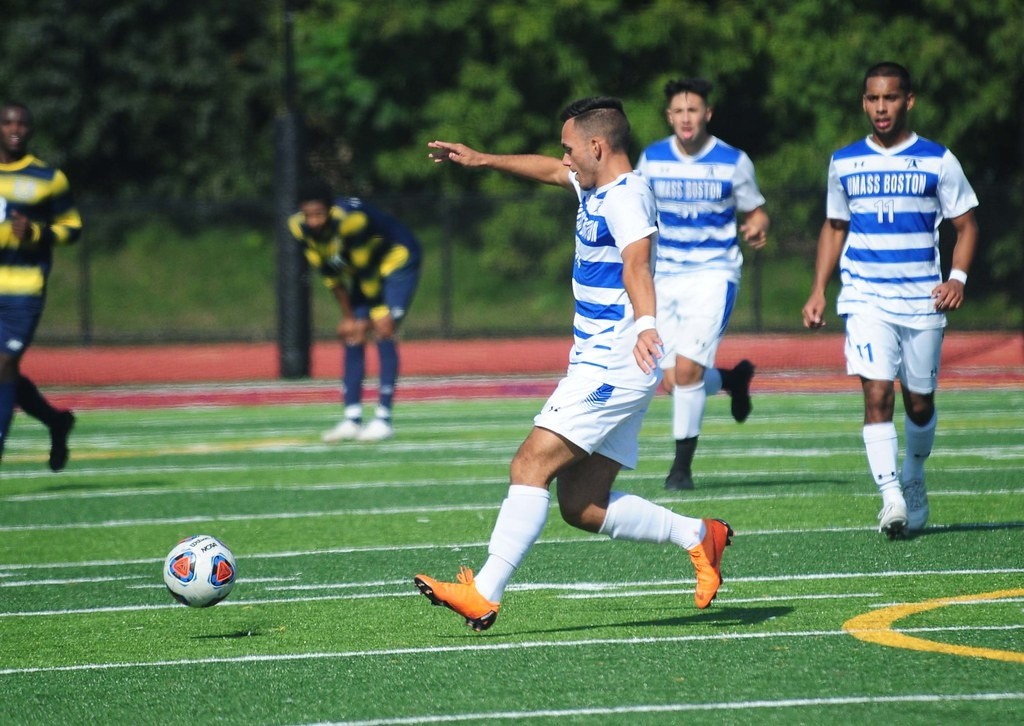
[49,410,77,471]
[323,420,362,442]
[358,420,392,441]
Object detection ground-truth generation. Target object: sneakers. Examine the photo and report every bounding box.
[901,463,929,533]
[688,519,734,609]
[414,566,500,632]
[878,500,907,537]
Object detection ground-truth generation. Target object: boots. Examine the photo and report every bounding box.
[664,434,699,490]
[717,358,754,424]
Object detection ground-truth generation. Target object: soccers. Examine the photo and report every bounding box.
[162,533,238,610]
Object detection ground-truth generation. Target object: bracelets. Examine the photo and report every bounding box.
[947,268,968,284]
[633,314,657,335]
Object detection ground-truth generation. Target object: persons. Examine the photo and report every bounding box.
[801,61,980,536]
[0,101,86,473]
[414,95,733,632]
[287,183,424,445]
[634,80,770,491]
